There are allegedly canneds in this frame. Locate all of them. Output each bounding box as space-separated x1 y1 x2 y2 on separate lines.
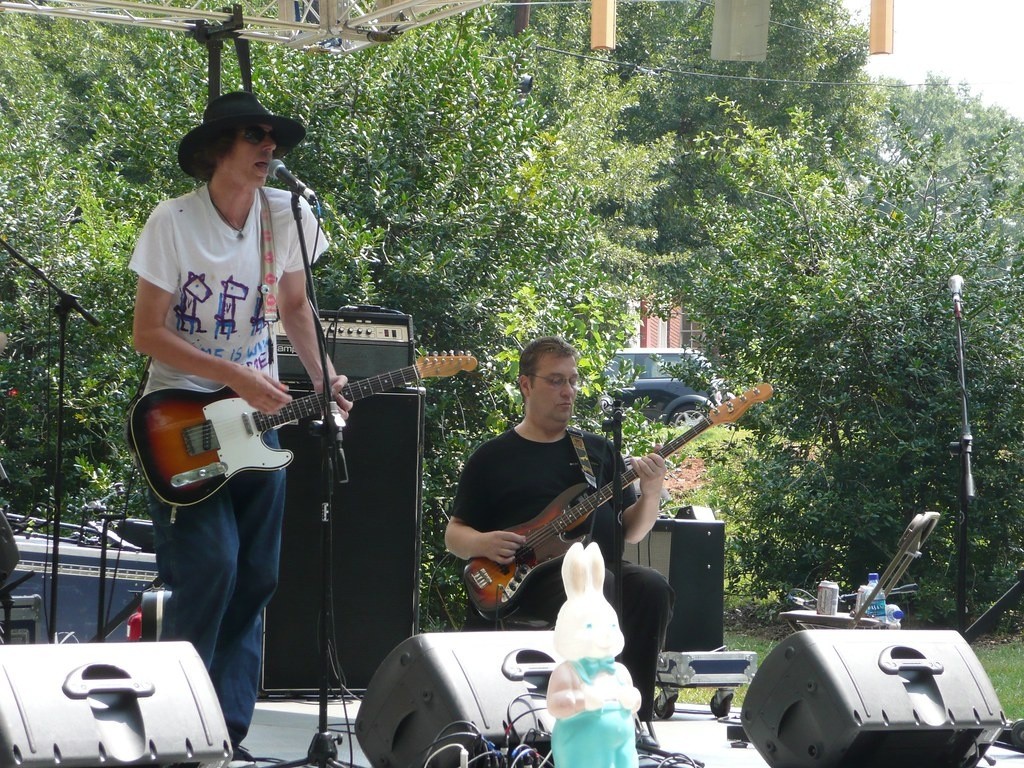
817 581 839 615
856 585 871 617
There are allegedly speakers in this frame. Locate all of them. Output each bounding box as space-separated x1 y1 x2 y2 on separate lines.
258 381 426 699
353 629 561 768
622 505 724 652
0 640 233 768
739 628 1007 768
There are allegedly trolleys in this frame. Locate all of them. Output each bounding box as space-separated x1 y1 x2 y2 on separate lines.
653 646 758 718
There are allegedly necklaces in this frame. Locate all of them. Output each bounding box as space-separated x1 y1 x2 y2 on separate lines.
210 198 254 238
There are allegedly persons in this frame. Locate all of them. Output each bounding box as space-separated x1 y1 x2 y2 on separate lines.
127 92 352 761
445 337 677 748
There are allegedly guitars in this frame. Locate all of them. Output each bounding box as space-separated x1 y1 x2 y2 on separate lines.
462 381 776 623
125 348 479 511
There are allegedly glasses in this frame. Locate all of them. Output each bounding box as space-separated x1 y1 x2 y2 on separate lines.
240 125 278 145
527 372 578 391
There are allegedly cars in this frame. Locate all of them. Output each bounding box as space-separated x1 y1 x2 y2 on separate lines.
601 348 723 430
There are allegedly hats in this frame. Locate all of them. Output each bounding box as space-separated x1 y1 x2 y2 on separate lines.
178 91 306 182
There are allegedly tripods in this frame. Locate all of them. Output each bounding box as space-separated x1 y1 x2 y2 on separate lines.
260 193 363 768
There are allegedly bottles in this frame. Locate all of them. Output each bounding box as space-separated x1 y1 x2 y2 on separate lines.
884 602 903 625
860 573 886 627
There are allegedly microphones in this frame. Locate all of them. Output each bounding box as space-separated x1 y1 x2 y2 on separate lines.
598 394 613 409
267 159 317 202
948 274 964 303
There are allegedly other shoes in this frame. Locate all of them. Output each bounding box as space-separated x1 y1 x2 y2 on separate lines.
232 742 255 762
